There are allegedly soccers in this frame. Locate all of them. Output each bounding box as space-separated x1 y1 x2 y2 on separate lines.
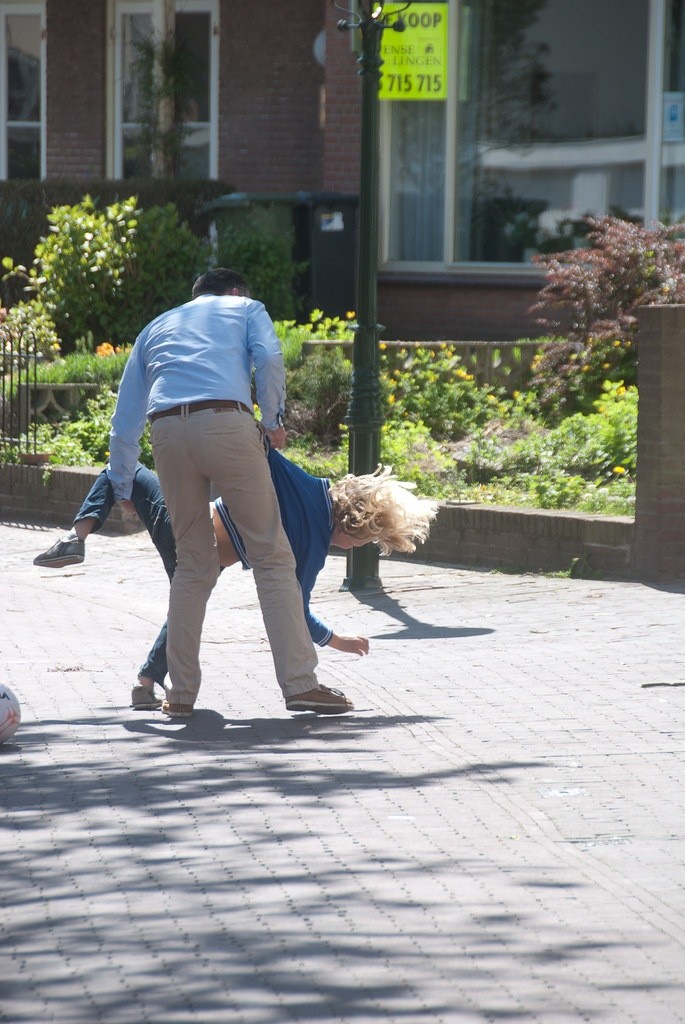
0 680 22 747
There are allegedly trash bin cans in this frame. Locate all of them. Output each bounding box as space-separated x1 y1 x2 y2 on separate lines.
197 190 363 324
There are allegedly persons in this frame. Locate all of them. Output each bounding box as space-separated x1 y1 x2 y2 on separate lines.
108 267 354 716
33 431 436 709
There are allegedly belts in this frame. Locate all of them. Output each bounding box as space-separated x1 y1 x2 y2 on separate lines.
151 400 251 423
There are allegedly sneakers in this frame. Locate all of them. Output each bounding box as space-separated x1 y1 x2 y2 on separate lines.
285 684 355 715
33 537 85 568
162 699 193 718
132 686 163 709
